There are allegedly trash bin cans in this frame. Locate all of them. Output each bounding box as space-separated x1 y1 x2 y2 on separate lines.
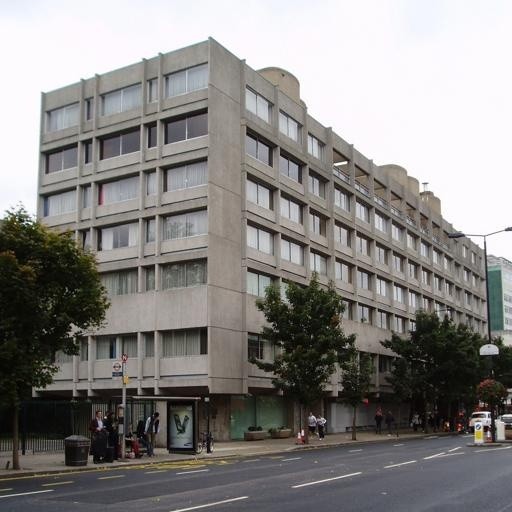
64 435 92 466
495 419 505 440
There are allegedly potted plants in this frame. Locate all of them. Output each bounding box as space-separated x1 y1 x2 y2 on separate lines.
268 425 292 438
244 426 266 441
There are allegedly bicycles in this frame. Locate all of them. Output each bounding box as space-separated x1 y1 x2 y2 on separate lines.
195 432 213 455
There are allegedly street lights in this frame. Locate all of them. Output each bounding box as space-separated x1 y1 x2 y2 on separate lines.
204 396 211 454
447 226 512 442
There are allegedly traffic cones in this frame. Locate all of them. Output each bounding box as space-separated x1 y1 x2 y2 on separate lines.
485 428 493 444
296 432 303 445
301 429 305 442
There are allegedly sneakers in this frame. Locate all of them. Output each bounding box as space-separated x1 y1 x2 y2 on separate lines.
93 459 103 464
317 433 324 440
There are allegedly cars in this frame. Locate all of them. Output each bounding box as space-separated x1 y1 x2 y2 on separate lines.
469 412 492 430
501 413 512 430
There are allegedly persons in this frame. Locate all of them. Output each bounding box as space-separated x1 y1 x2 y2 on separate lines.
136 416 151 456
103 410 109 429
315 413 326 440
89 410 107 463
374 407 477 434
107 411 120 460
143 411 161 456
308 411 317 439
